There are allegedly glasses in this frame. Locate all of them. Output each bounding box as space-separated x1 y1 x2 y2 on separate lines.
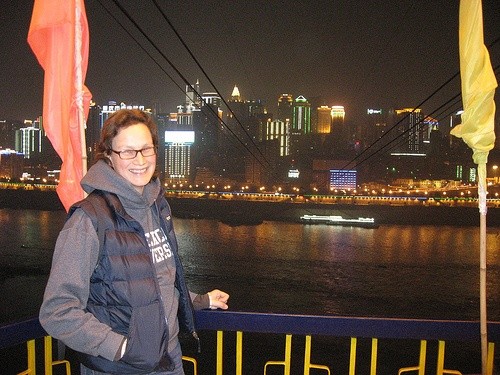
110 147 158 160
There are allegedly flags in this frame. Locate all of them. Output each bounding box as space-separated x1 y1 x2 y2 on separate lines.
24 1 94 215
449 0 499 163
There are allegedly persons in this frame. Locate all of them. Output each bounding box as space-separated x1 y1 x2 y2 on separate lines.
38 110 231 375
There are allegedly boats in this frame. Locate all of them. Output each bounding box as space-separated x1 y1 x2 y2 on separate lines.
298 214 379 229
